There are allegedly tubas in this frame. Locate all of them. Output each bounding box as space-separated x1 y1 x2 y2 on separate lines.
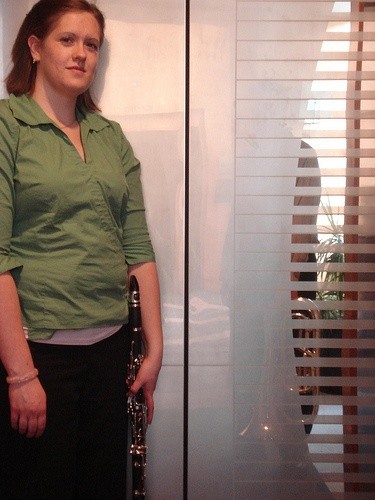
288 296 320 424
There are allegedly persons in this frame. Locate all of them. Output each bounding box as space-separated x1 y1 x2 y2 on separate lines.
291 139 322 436
1 2 162 500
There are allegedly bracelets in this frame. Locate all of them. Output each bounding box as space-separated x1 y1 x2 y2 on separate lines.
6 369 39 385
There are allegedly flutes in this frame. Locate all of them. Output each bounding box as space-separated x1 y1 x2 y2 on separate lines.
126 274 148 500
